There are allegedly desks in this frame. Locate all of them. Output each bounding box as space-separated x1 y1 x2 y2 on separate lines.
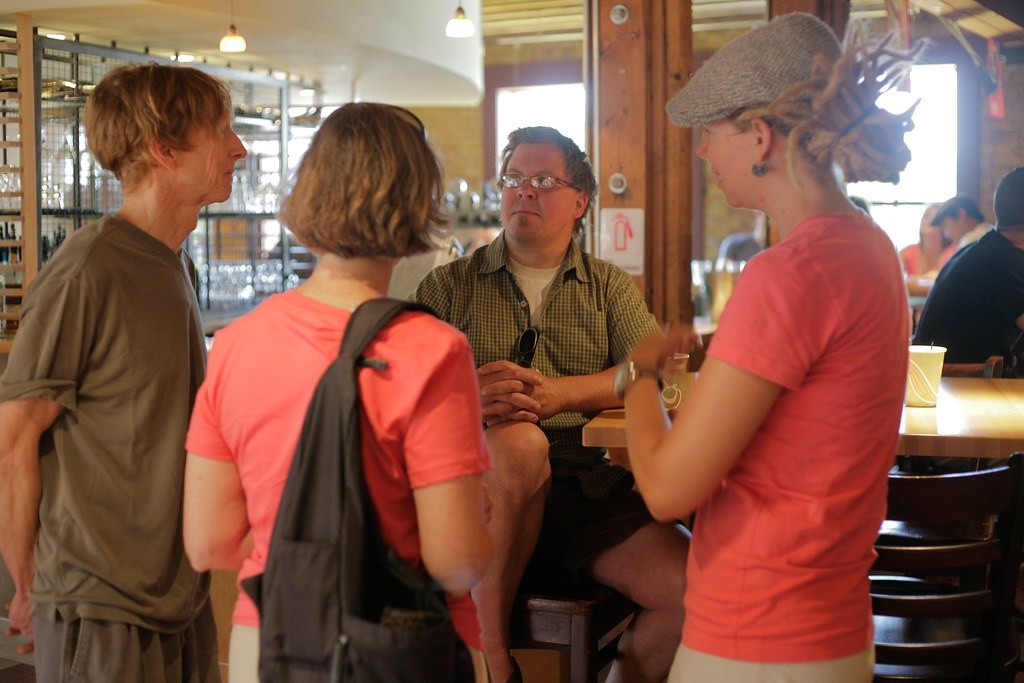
584 369 1024 462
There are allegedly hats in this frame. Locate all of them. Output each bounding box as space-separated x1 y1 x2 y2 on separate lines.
666 13 841 127
993 166 1024 230
930 197 974 226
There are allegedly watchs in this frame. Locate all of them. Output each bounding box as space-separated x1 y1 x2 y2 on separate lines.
614 360 663 400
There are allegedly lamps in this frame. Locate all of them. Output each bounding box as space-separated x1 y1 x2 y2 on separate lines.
443 0 476 40
218 0 247 53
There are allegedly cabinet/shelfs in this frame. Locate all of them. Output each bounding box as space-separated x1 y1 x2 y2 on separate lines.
0 23 315 353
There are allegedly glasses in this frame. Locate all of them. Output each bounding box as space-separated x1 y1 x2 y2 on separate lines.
517 327 540 369
501 174 581 191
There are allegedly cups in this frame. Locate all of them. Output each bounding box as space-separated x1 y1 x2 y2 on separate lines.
658 351 690 406
904 345 947 406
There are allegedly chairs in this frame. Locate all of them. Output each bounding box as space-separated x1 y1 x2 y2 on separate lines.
864 448 1024 683
509 578 636 683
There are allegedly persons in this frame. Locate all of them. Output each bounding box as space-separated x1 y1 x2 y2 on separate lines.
184 105 493 683
408 125 686 682
717 166 1024 643
614 13 912 683
0 62 250 682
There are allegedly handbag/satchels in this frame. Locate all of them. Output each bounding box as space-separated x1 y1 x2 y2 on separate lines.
240 297 474 683
